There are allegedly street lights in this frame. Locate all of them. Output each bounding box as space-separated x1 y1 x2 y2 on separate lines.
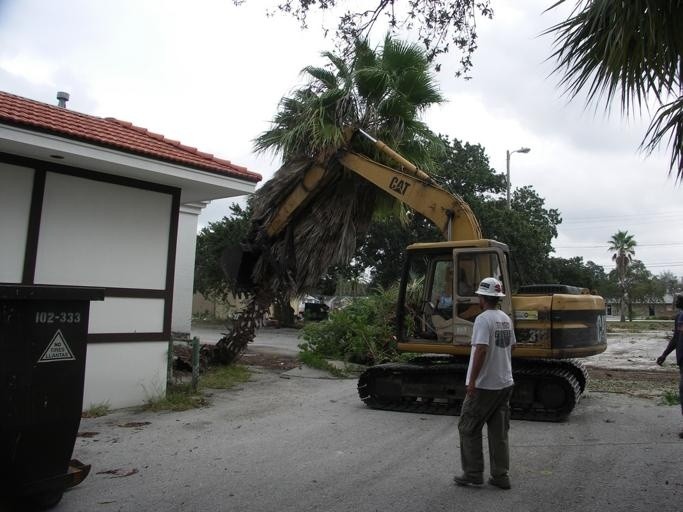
503 147 530 209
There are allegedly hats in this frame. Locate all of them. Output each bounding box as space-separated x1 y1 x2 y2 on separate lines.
472 277 507 297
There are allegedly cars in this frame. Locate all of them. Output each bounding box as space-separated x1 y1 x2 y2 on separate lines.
262 316 279 327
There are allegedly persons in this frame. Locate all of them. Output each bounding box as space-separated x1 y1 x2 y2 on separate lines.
453 278 517 490
656 294 683 438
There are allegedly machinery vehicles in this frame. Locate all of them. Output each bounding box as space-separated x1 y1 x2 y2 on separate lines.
217 117 608 422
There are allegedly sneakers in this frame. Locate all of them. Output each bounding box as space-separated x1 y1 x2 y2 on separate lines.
486 474 513 491
453 469 486 487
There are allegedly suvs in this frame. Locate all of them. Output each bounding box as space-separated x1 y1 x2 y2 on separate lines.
297 299 319 319
